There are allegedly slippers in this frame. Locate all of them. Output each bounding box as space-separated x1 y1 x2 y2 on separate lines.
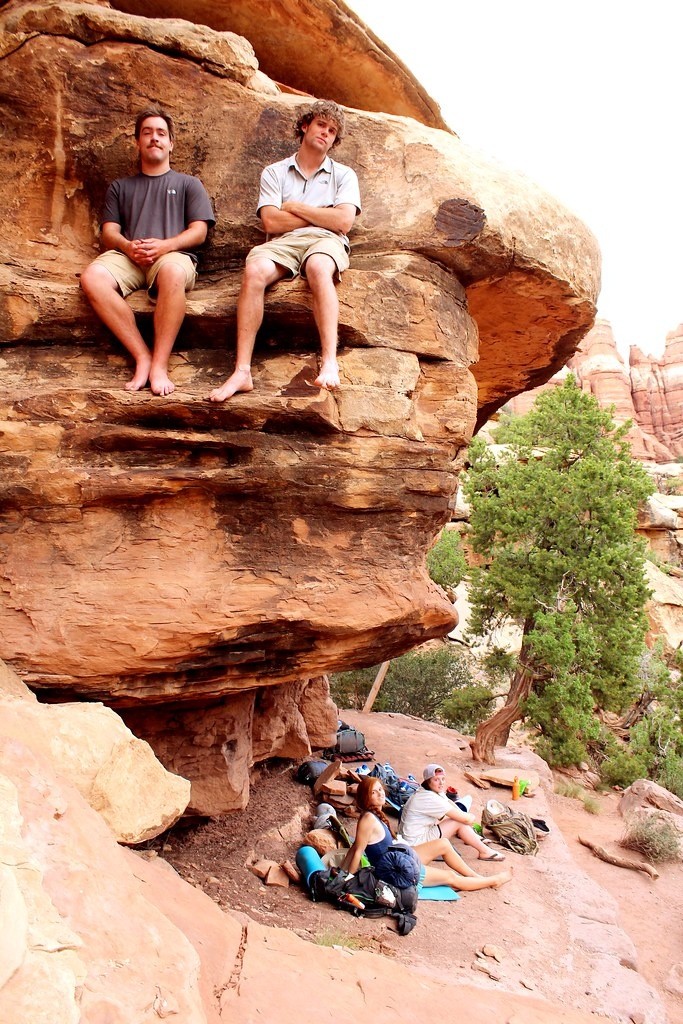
477 851 506 861
464 833 492 845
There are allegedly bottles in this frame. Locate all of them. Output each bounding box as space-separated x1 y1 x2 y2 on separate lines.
384 763 394 773
408 773 415 781
346 893 365 910
360 763 369 775
513 776 519 799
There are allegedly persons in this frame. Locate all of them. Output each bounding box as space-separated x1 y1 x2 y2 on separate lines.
80 105 217 394
210 99 362 402
341 777 515 891
398 764 506 861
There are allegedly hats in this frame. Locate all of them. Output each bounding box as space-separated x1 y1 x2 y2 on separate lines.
423 764 445 781
